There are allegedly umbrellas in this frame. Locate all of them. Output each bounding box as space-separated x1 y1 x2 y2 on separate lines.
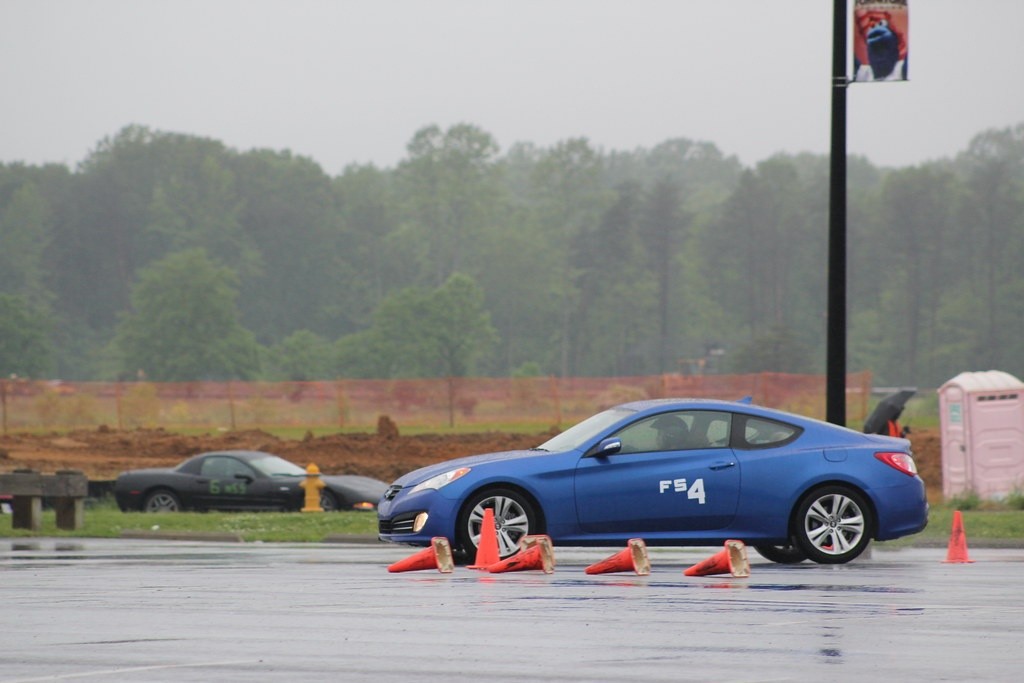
862 388 915 434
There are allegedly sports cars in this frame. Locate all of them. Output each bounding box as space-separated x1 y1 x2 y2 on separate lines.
115 448 390 517
379 390 930 565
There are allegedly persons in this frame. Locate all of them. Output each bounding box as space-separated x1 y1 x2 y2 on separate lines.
648 414 688 451
879 407 910 439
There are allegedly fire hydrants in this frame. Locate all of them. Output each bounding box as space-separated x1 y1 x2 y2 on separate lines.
298 462 326 514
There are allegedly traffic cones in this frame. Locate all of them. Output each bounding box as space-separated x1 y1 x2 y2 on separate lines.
937 508 977 565
520 535 557 568
684 540 751 579
386 536 454 573
466 507 501 569
485 537 555 575
583 537 651 576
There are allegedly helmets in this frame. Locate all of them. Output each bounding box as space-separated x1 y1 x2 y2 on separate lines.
650 414 690 440
